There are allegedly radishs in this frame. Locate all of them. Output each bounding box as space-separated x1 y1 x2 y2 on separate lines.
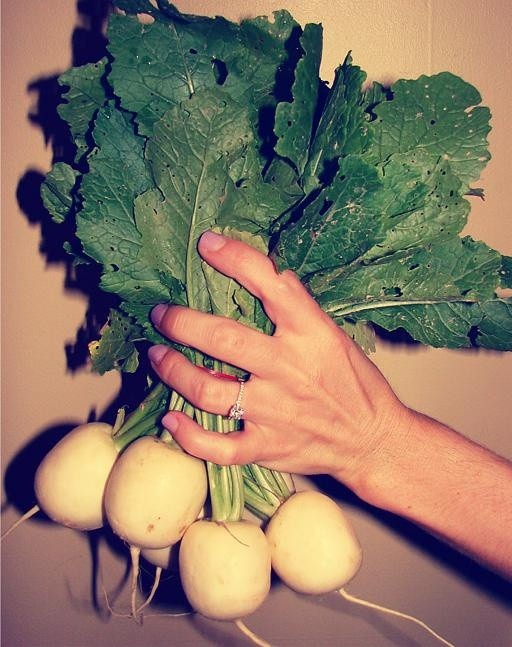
104 436 209 619
178 519 270 647
0 421 120 542
264 491 454 647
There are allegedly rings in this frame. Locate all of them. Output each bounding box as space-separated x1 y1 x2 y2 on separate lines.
225 379 246 423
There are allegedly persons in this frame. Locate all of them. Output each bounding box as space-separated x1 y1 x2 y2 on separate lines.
146 229 512 586
